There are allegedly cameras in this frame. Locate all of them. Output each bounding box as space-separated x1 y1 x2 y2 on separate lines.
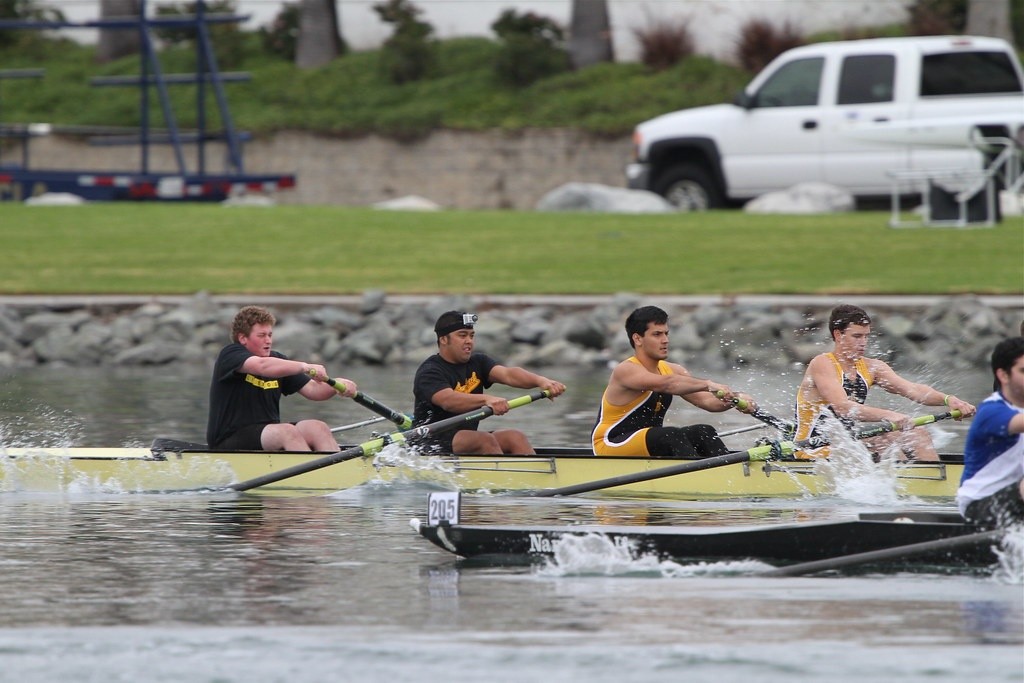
462 313 478 325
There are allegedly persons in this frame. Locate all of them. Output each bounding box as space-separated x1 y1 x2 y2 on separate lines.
205 304 358 452
411 309 567 455
591 305 760 457
956 337 1024 528
792 304 977 462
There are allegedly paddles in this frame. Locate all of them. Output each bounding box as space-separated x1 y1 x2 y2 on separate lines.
409 405 976 496
563 522 1023 578
306 367 418 431
127 383 568 491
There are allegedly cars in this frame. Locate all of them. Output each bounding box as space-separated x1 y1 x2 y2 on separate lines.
623 35 1024 215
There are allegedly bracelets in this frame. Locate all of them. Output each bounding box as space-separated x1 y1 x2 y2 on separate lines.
944 395 950 406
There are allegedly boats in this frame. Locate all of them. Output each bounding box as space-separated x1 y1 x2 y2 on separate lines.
1 434 963 500
407 508 997 572
